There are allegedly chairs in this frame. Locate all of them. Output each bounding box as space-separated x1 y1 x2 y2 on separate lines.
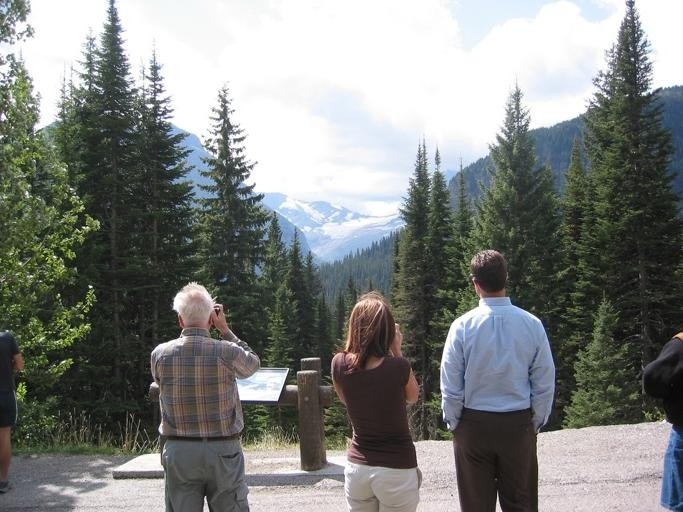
1 481 10 492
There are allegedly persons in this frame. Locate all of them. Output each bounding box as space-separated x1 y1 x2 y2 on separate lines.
331 288 422 512
642 332 683 512
0 332 25 493
150 281 261 512
438 248 557 511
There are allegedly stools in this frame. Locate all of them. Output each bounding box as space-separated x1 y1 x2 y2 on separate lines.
166 435 242 441
